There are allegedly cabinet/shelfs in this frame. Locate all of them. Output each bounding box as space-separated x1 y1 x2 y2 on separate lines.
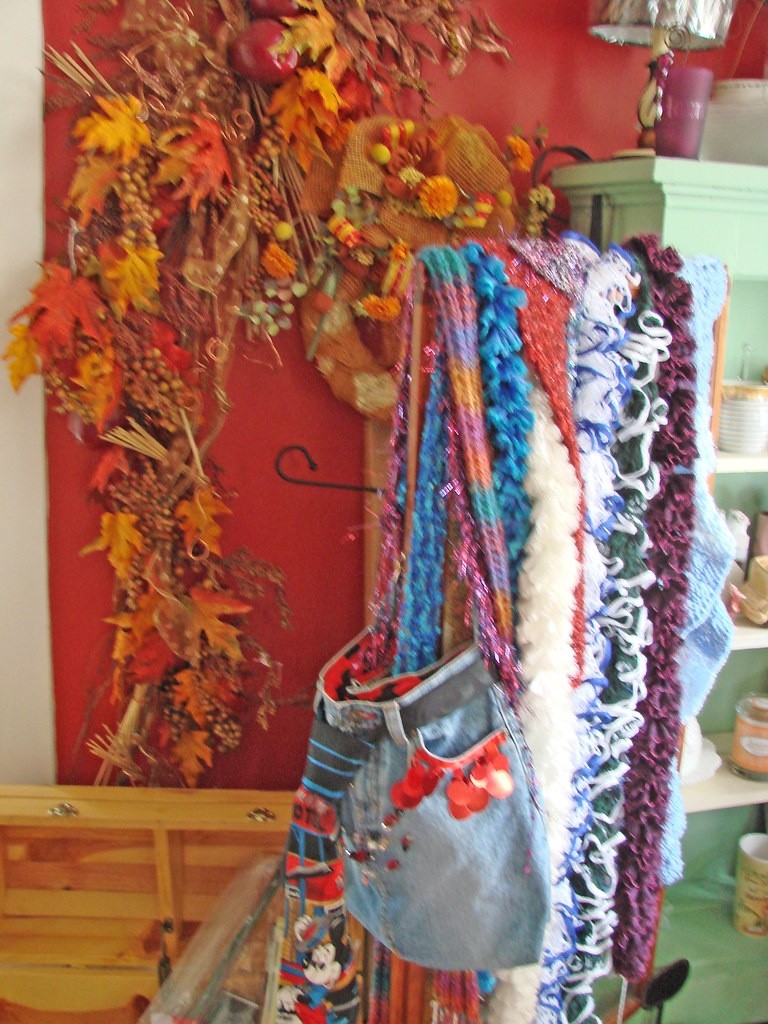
551 152 768 1024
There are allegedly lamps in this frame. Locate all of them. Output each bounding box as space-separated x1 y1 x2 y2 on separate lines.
587 0 738 159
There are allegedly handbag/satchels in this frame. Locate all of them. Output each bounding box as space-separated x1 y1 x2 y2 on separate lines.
300 111 520 423
276 621 551 1024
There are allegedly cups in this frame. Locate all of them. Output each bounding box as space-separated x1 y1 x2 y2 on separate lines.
718 379 768 455
754 511 768 558
655 64 714 159
733 833 768 938
715 77 766 102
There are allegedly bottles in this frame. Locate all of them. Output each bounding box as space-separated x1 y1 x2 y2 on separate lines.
728 511 751 571
729 693 768 782
461 968 499 1024
